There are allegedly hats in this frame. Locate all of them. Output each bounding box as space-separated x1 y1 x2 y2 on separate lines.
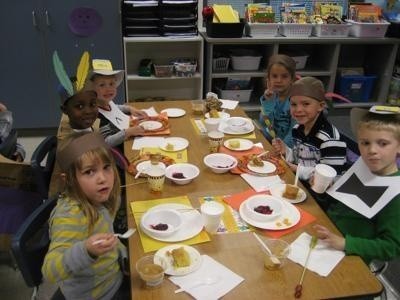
88 59 125 88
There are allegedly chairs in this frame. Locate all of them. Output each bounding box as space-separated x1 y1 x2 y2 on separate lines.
32 134 60 197
338 129 362 174
10 197 62 299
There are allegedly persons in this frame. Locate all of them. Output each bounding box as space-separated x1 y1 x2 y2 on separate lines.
47 75 128 206
259 53 297 143
307 113 400 266
40 131 131 299
270 76 347 181
88 58 148 148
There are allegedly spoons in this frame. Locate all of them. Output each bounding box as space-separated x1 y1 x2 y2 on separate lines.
174 276 221 294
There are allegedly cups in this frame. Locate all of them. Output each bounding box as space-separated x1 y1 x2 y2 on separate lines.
136 256 168 286
261 238 290 271
312 162 338 195
191 100 205 113
200 201 224 233
147 167 165 192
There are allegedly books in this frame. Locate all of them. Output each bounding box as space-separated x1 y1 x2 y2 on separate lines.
211 1 384 26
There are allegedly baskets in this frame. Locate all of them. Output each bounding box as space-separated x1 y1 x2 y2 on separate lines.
213 58 230 71
245 19 391 37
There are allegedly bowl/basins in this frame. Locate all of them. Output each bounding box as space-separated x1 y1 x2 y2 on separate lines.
204 153 238 175
245 196 282 221
145 210 183 234
166 163 202 185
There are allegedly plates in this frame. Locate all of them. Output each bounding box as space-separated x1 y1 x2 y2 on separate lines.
240 194 299 232
205 110 255 153
136 161 165 176
269 182 306 204
247 159 276 172
155 244 202 277
138 107 189 151
141 203 203 242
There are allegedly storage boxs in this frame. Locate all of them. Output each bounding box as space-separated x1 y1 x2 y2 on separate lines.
207 19 244 38
344 18 390 37
282 53 309 70
339 73 378 102
216 86 254 103
229 54 263 71
312 22 350 36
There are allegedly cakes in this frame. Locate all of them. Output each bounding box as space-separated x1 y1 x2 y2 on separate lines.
171 246 190 268
165 141 174 150
209 108 220 119
228 140 240 148
282 185 299 200
254 157 265 168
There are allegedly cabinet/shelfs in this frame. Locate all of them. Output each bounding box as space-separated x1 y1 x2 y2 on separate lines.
202 29 400 113
123 37 203 100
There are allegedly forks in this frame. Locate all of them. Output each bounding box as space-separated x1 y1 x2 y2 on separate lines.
93 227 137 246
